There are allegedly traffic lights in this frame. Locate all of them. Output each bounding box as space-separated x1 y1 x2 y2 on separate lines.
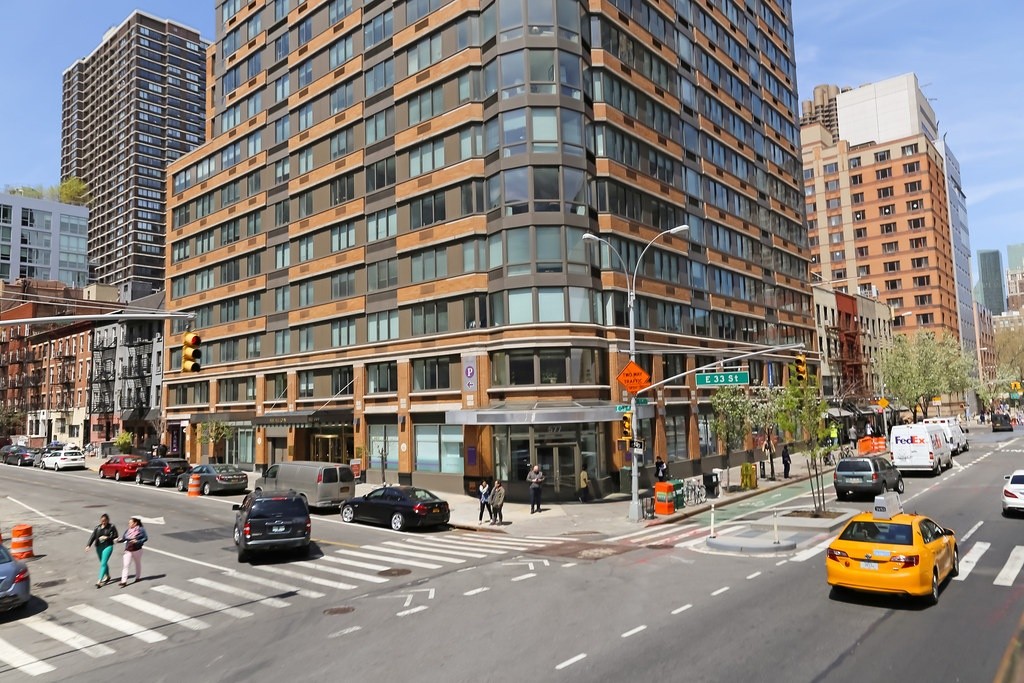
180 329 203 374
621 413 632 438
794 354 808 383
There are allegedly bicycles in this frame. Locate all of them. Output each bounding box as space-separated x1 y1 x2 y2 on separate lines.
684 479 709 507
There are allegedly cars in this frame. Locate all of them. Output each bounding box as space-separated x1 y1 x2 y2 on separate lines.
338 485 452 532
0 443 87 471
833 456 904 502
1001 469 1024 518
231 488 312 563
98 454 148 481
175 463 249 496
135 458 193 487
824 491 959 607
0 545 32 617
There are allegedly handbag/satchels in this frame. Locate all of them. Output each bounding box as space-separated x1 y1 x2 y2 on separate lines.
658 468 663 477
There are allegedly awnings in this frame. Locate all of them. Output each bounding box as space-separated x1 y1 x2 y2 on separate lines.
251 409 353 428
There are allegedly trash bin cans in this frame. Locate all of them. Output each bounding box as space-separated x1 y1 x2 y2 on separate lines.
741 463 758 489
654 481 675 514
702 472 719 497
670 480 685 509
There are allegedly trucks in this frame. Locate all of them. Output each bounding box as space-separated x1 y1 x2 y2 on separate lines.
254 460 355 515
923 417 970 455
890 422 954 476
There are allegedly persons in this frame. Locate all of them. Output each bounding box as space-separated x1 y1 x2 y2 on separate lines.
579 463 590 504
830 421 874 450
761 438 775 462
478 480 493 524
655 456 667 481
117 518 148 586
526 465 545 514
489 480 505 525
782 445 792 478
85 513 118 587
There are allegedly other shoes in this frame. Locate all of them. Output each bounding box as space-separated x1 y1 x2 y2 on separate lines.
531 508 534 514
496 522 503 525
579 497 583 504
134 577 139 583
118 582 126 586
478 520 481 525
489 521 496 525
102 576 110 582
537 508 541 512
95 584 101 588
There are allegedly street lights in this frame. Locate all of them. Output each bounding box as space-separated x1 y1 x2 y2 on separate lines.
875 310 913 444
581 225 690 524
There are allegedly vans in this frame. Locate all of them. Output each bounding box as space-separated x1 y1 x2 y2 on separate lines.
992 413 1013 432
